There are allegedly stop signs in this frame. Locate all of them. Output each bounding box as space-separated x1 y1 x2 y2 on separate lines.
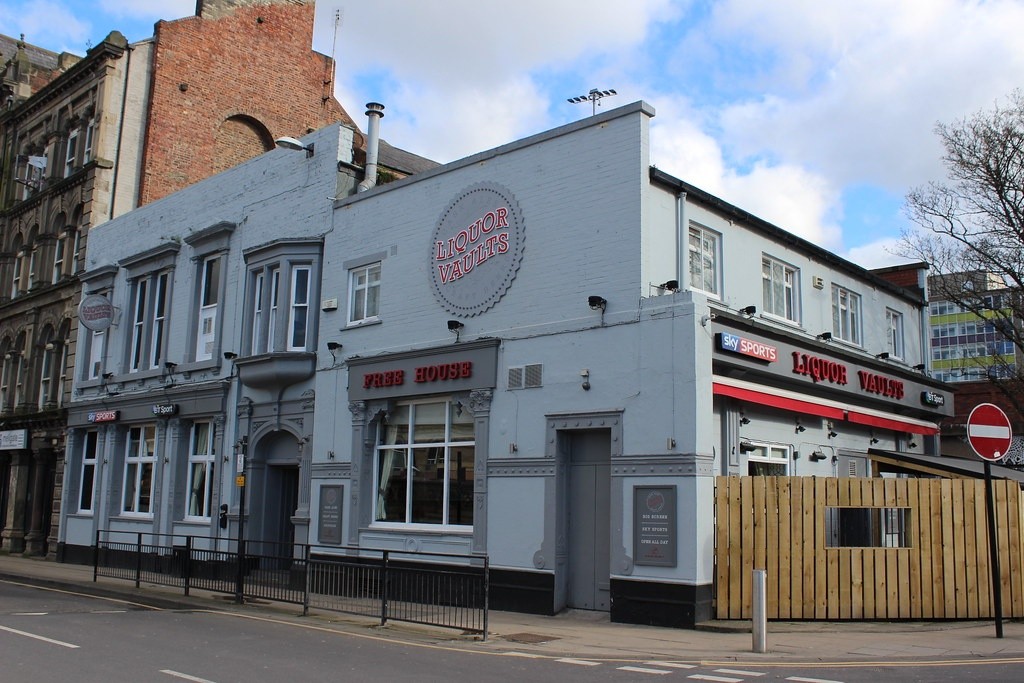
966 403 1012 461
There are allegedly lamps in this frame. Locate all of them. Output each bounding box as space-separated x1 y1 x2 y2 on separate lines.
660 280 679 291
740 418 750 426
447 320 464 333
165 361 178 369
588 296 607 310
818 333 831 342
224 352 237 359
102 373 114 380
796 425 806 434
913 364 925 371
740 306 756 317
812 451 826 460
876 352 889 360
870 438 879 444
910 443 917 448
275 136 314 152
327 342 343 351
828 432 837 439
740 442 756 454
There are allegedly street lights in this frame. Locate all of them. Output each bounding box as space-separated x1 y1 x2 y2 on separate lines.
233 435 248 603
567 89 617 117
